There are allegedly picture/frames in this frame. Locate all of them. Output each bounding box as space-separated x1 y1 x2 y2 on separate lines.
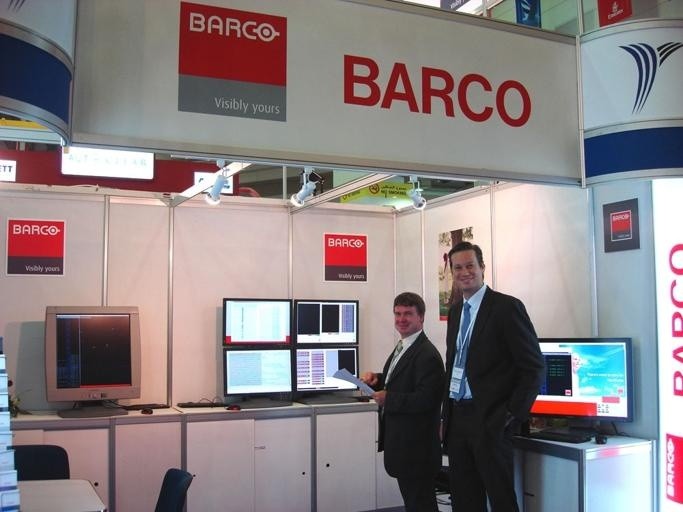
601 197 641 254
321 230 370 285
4 215 66 278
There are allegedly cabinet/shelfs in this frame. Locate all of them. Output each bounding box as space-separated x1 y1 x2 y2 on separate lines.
314 410 379 511
115 420 182 512
515 425 658 512
12 428 43 443
42 428 112 511
253 415 314 512
185 419 253 512
377 449 406 509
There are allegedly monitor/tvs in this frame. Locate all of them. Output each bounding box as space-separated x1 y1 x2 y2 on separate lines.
528 338 633 435
294 299 358 345
46 306 141 418
293 345 359 405
223 349 293 408
222 298 293 349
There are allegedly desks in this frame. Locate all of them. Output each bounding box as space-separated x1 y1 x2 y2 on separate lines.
12 478 108 511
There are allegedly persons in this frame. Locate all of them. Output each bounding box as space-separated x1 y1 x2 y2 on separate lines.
362 290 446 511
442 247 543 511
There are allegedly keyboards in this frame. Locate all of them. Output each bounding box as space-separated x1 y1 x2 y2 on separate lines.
526 432 592 443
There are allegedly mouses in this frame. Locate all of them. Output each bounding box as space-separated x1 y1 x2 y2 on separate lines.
143 407 154 415
595 435 607 444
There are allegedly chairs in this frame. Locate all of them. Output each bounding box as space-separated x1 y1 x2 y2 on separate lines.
7 444 70 479
154 466 193 511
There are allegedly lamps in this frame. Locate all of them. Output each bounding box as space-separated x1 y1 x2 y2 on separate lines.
205 156 429 211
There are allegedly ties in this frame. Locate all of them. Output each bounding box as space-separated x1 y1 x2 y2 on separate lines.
379 340 403 418
451 301 471 403
